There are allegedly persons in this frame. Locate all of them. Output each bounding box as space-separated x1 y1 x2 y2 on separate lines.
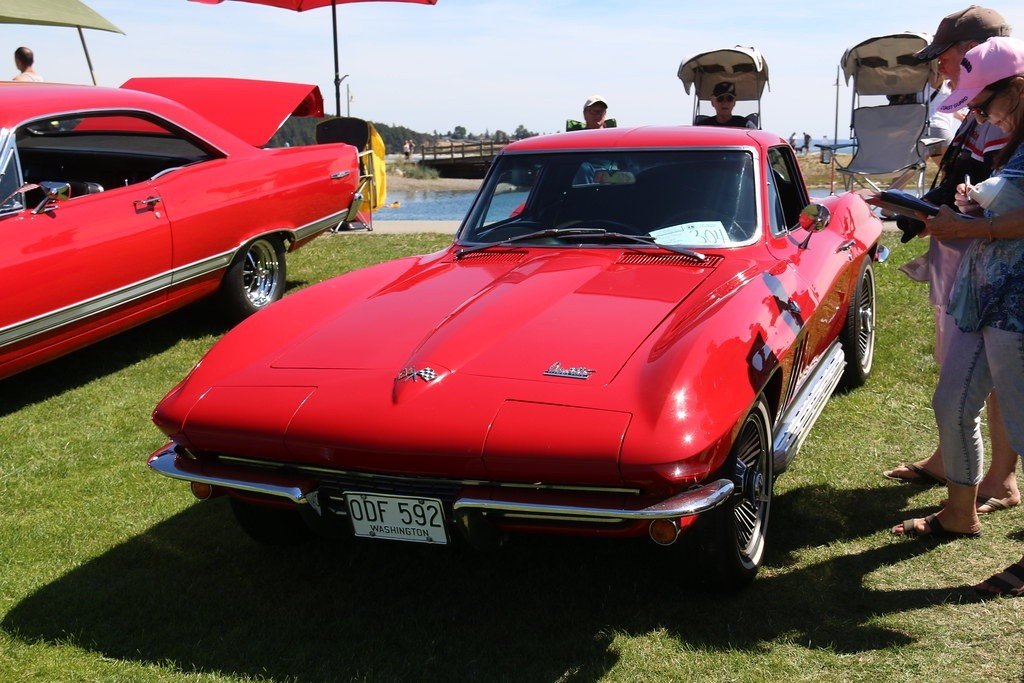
789 132 796 149
892 35 1024 597
403 139 411 158
882 4 1021 515
696 82 757 130
801 132 810 153
408 140 415 156
12 47 44 82
571 97 618 186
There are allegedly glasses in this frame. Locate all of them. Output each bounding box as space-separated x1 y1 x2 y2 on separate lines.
968 89 997 118
585 110 606 115
713 95 735 102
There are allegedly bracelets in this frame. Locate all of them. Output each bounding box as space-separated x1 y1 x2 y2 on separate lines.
989 217 993 243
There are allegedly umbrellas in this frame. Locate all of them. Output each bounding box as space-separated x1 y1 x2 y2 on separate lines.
188 0 438 117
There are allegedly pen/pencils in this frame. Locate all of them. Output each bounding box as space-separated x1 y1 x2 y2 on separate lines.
964 174 971 203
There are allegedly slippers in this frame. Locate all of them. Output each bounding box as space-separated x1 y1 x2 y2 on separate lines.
882 464 946 484
941 492 1010 513
891 513 982 537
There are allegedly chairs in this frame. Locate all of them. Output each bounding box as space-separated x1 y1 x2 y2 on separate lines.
566 118 616 132
812 58 932 214
692 65 763 130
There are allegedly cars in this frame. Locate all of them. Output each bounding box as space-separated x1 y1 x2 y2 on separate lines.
0 77 362 389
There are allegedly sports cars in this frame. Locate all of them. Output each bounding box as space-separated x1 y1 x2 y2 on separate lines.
142 123 892 595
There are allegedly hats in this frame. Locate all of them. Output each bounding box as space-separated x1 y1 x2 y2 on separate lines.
913 3 1014 67
583 95 608 109
935 36 1024 113
712 82 736 97
